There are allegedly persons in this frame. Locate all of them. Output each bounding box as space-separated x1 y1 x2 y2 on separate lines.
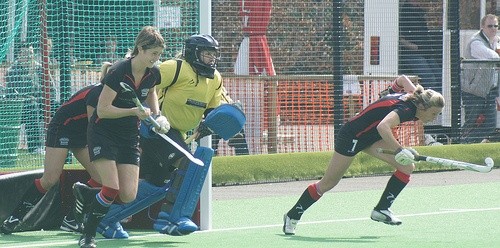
72 25 171 248
398 0 442 92
96 34 246 239
0 37 58 154
460 14 500 144
283 75 445 236
0 57 119 235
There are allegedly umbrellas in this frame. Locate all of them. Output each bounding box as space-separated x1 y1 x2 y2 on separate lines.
236 0 280 133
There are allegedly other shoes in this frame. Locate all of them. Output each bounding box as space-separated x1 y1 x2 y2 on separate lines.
33 149 45 156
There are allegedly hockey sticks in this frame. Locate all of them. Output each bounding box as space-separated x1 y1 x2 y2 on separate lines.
167 131 199 160
151 127 204 167
120 81 160 129
376 148 494 173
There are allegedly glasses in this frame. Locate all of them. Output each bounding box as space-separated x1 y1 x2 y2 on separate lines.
484 23 499 29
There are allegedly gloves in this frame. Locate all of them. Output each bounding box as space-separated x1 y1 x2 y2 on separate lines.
395 147 415 165
154 116 170 134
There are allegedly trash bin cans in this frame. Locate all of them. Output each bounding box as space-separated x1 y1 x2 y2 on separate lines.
0 98 26 168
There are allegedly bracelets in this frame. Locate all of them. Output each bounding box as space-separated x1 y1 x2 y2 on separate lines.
153 110 162 119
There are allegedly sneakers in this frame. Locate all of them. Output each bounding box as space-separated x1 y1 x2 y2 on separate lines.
1 216 20 236
71 182 89 222
371 208 402 225
283 213 299 235
60 216 85 236
78 232 96 248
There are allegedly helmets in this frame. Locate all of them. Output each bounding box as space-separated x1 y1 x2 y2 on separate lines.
185 34 220 79
